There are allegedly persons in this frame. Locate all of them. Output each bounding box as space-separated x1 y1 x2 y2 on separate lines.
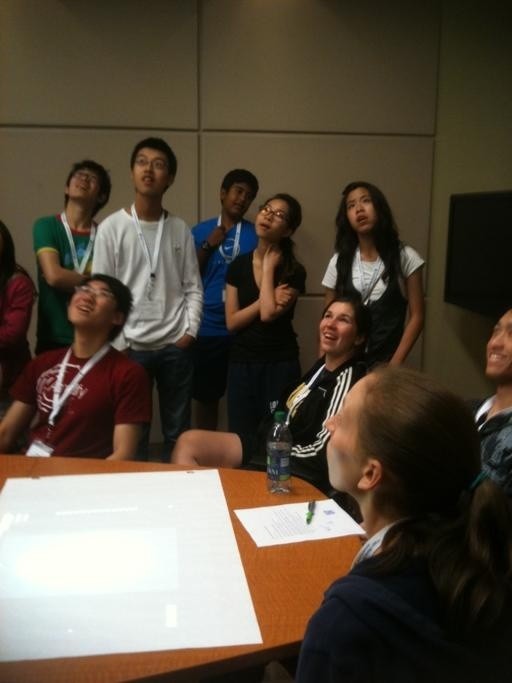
319 181 425 376
263 362 510 682
171 293 369 520
34 159 111 353
223 191 308 435
0 272 153 462
189 169 259 432
92 133 204 463
472 303 512 478
0 219 36 455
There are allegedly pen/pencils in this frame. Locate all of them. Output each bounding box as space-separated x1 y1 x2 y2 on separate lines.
306 501 316 524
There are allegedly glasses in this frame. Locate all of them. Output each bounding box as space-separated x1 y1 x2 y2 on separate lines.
73 171 101 181
260 206 286 218
72 284 117 300
136 159 167 168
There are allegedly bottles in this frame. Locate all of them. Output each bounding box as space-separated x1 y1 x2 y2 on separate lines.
266 412 291 494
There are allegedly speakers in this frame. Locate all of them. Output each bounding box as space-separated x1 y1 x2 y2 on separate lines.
444 191 512 318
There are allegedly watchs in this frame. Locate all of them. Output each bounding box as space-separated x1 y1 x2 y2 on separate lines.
201 240 212 252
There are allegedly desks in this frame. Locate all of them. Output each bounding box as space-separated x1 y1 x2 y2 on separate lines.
1 453 369 683
